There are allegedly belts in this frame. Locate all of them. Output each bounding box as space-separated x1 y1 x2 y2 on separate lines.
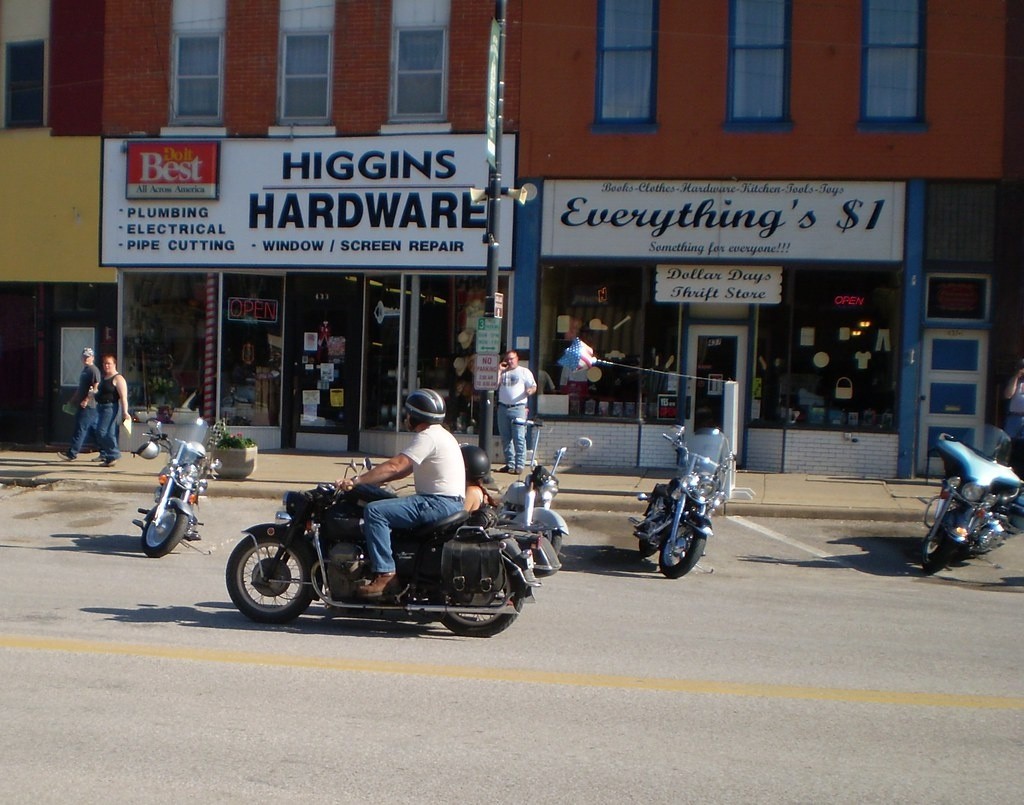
1009 412 1024 416
500 403 525 408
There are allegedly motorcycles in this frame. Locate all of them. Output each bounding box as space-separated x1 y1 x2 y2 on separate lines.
224 417 564 638
627 424 735 580
129 408 222 559
917 422 1024 576
499 418 570 557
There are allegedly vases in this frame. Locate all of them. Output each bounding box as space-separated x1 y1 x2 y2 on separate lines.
213 446 258 478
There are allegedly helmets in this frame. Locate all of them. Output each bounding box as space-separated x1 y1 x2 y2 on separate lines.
405 388 446 426
135 439 161 459
460 446 491 484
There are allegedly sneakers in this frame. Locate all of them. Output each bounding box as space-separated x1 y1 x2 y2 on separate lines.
359 573 398 596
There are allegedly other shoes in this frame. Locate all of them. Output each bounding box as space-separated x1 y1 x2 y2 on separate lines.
515 467 523 474
57 451 73 461
100 459 118 467
500 466 515 475
92 455 107 462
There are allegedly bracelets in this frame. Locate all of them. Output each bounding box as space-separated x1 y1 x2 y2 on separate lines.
351 476 360 486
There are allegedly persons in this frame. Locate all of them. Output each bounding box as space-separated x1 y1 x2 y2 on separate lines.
335 389 466 595
1004 357 1024 439
538 370 588 413
57 346 100 462
460 445 490 513
497 351 537 476
90 354 131 466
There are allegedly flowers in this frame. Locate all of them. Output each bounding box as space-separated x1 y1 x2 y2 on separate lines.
217 432 255 450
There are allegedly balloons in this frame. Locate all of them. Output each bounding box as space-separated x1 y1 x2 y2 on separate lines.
557 337 598 373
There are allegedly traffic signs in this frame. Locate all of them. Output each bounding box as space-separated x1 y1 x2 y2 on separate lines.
494 291 504 319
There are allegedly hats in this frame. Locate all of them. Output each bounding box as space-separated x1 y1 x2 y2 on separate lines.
82 347 94 357
